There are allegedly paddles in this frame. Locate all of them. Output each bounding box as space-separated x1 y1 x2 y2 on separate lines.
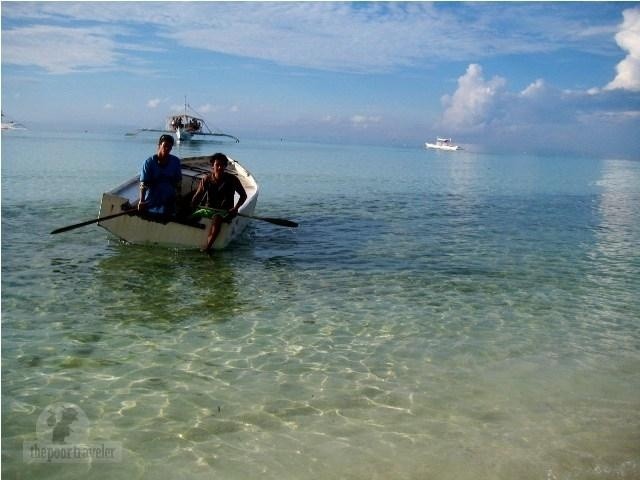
198 204 298 228
50 206 139 235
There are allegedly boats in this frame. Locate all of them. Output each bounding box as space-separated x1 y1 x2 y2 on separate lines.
97 154 259 251
125 97 240 142
425 136 461 151
1 111 29 131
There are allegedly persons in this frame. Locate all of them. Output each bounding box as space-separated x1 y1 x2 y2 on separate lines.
137 135 183 222
187 153 247 251
170 117 202 137
